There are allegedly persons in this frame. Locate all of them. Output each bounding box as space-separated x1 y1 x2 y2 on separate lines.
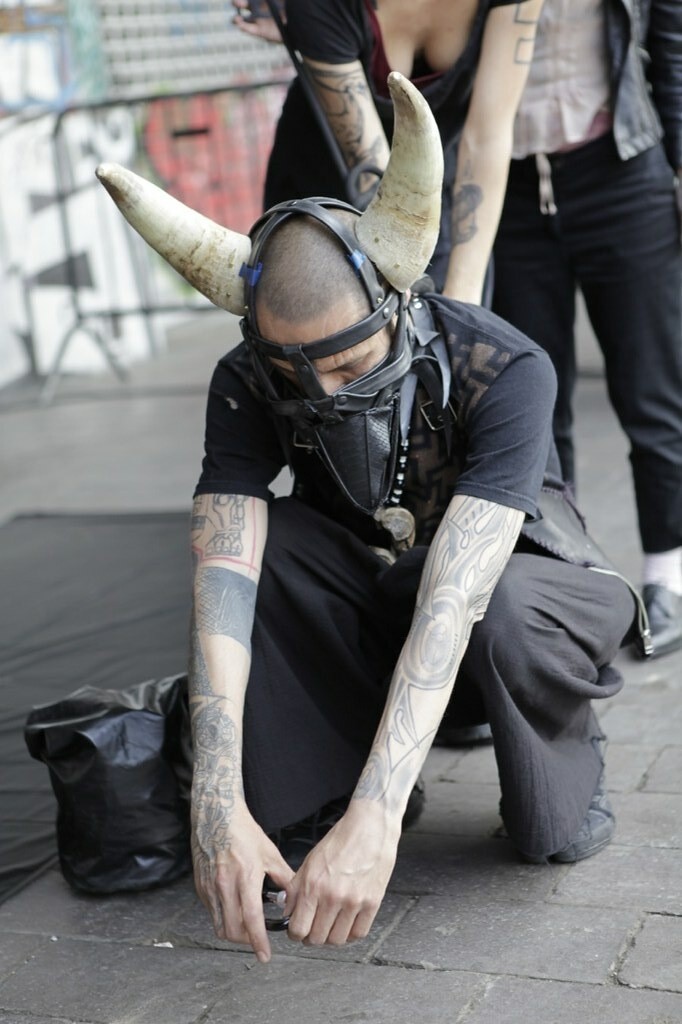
92 72 657 963
228 0 682 659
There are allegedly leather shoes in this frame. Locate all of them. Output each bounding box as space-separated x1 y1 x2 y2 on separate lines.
637 584 682 663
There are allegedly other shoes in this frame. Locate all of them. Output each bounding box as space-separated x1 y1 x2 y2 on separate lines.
257 771 424 899
546 761 616 864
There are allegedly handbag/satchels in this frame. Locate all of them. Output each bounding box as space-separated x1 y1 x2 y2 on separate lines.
24 669 193 896
